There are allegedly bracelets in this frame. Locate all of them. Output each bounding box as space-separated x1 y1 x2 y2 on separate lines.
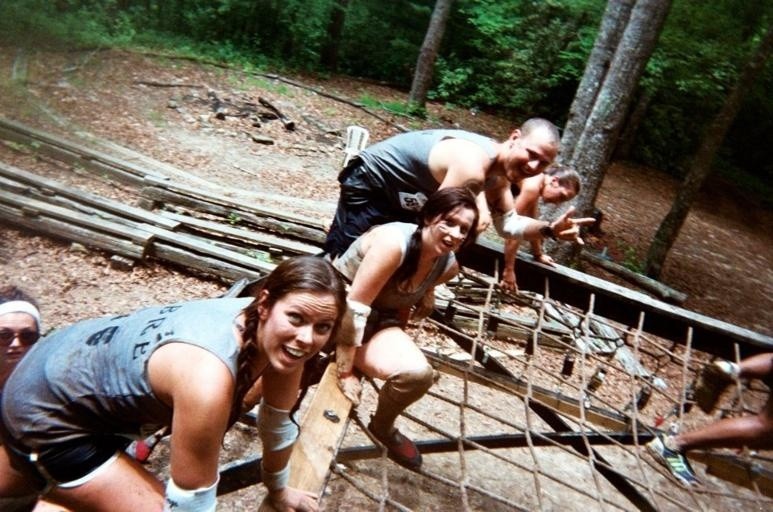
258 459 290 490
542 220 555 240
336 363 353 381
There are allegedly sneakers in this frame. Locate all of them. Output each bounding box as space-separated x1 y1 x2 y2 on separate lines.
638 432 704 488
366 415 422 468
693 359 742 415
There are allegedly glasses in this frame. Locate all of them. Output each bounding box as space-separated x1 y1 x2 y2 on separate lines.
0 331 38 345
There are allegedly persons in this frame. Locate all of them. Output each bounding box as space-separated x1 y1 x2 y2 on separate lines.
0 255 348 512
320 116 597 259
0 283 85 512
455 171 584 296
643 352 773 490
239 183 479 472
